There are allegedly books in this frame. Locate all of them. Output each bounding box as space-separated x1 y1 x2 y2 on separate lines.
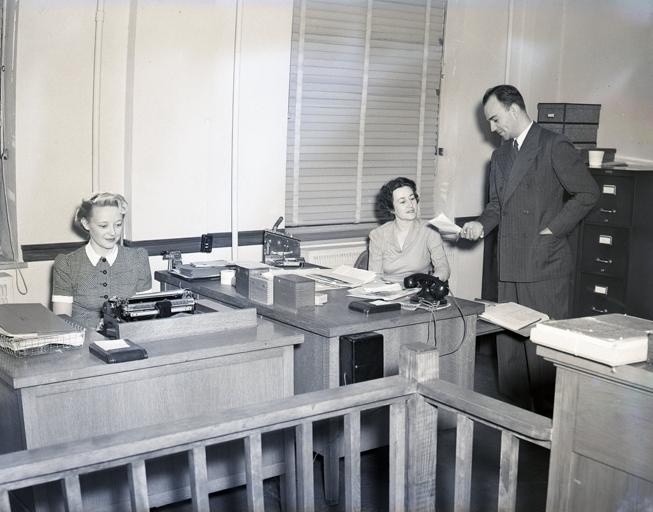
169 257 234 281
268 262 419 312
529 310 652 368
470 295 551 341
1 302 87 360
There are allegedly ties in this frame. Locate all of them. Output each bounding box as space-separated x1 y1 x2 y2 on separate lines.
511 140 519 164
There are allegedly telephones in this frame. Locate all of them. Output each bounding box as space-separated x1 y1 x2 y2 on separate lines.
404 273 449 307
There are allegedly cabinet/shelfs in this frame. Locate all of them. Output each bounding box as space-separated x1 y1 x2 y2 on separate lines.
480 156 652 327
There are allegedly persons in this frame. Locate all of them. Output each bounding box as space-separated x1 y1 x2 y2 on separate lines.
365 174 451 282
458 83 600 419
50 191 153 330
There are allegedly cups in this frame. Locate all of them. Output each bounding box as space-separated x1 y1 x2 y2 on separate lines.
587 150 604 168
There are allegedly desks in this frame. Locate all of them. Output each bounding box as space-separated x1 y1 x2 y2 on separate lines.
1 301 306 510
536 332 653 512
155 255 486 458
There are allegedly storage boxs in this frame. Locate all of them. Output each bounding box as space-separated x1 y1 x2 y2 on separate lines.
570 142 597 160
535 100 601 126
537 121 599 145
272 274 316 312
232 261 269 297
